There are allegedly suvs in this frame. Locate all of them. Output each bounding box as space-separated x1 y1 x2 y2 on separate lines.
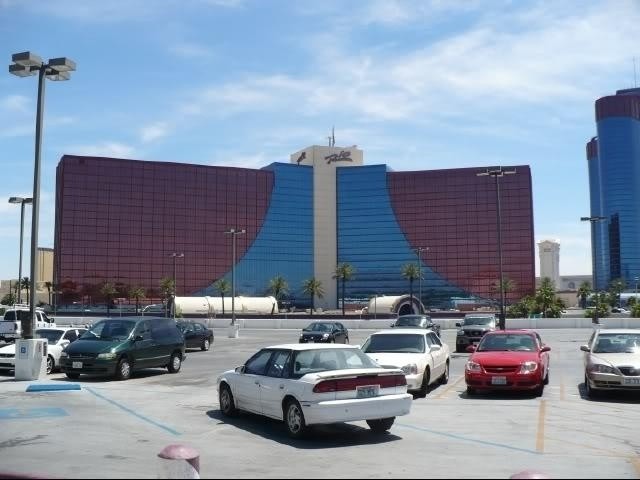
455 313 502 352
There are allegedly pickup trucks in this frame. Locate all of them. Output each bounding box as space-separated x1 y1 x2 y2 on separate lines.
0 305 57 341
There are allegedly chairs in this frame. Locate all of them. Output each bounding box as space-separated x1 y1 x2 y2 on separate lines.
598 339 640 352
110 328 127 337
281 360 336 377
318 325 327 331
193 326 200 331
488 338 533 347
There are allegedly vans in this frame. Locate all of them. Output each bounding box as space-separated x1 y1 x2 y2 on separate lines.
59 317 187 380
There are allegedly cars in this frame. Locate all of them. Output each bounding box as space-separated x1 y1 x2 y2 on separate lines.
217 344 411 437
349 329 449 398
0 326 101 374
299 321 349 343
175 321 214 352
463 330 552 395
391 314 440 339
581 328 640 397
128 303 161 312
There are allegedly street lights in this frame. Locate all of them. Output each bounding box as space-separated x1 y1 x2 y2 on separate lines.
5 52 83 341
633 277 640 305
169 253 185 297
580 215 610 311
7 196 34 303
477 166 518 331
411 245 430 313
223 228 247 324
